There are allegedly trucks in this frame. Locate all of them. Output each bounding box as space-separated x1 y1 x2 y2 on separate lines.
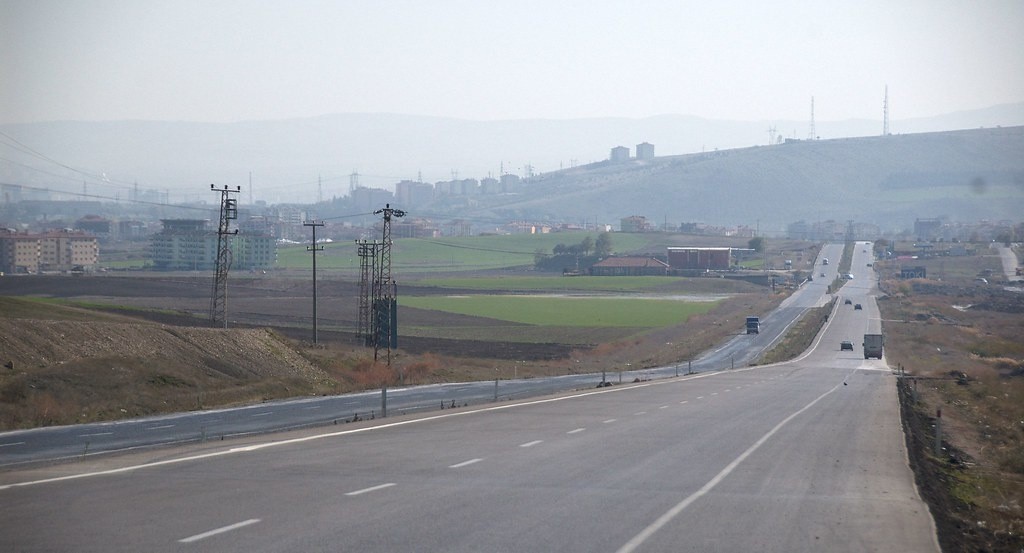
862 334 886 360
744 317 761 334
824 259 828 264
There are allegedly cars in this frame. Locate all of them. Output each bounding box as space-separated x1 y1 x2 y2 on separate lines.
840 341 855 350
821 273 826 277
845 299 853 304
855 304 863 310
974 277 988 284
863 249 866 252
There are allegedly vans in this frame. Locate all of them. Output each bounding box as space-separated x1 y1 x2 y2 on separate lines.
843 274 853 279
